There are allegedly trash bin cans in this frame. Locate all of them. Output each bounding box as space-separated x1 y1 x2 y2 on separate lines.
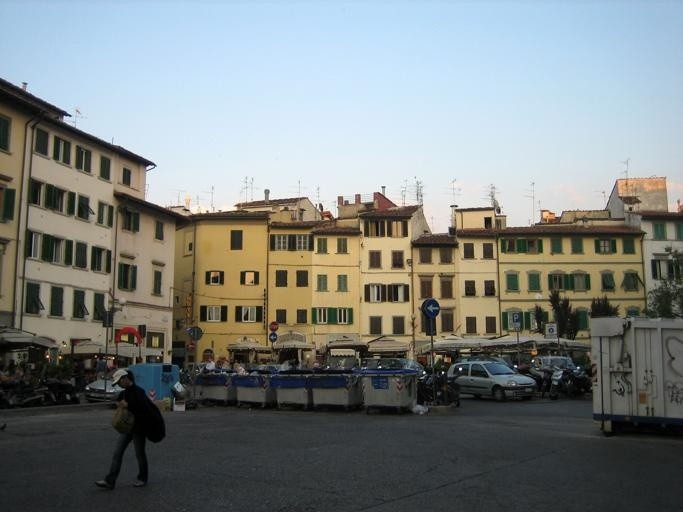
272 372 310 410
310 368 363 411
233 373 277 408
359 369 418 415
196 370 236 406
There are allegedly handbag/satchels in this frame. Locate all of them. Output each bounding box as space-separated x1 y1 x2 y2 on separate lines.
112 406 134 435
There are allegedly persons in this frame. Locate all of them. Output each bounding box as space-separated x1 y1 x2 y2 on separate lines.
196 356 308 376
93 368 148 490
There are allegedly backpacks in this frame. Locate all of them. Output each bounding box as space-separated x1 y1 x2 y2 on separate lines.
147 403 166 443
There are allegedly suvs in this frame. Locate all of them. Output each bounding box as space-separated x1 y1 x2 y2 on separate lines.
453 355 518 374
351 357 424 377
524 356 579 391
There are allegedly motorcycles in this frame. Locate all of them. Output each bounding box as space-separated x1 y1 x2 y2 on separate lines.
539 363 590 400
415 371 464 408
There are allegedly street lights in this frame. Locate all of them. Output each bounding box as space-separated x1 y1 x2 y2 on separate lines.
305 354 310 369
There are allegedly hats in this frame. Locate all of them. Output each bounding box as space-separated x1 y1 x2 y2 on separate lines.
112 370 128 387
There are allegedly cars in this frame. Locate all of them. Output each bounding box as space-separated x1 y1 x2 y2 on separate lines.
447 362 537 402
254 364 292 371
82 367 132 404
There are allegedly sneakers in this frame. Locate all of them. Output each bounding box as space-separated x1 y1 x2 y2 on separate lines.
95 480 114 488
133 480 144 487
